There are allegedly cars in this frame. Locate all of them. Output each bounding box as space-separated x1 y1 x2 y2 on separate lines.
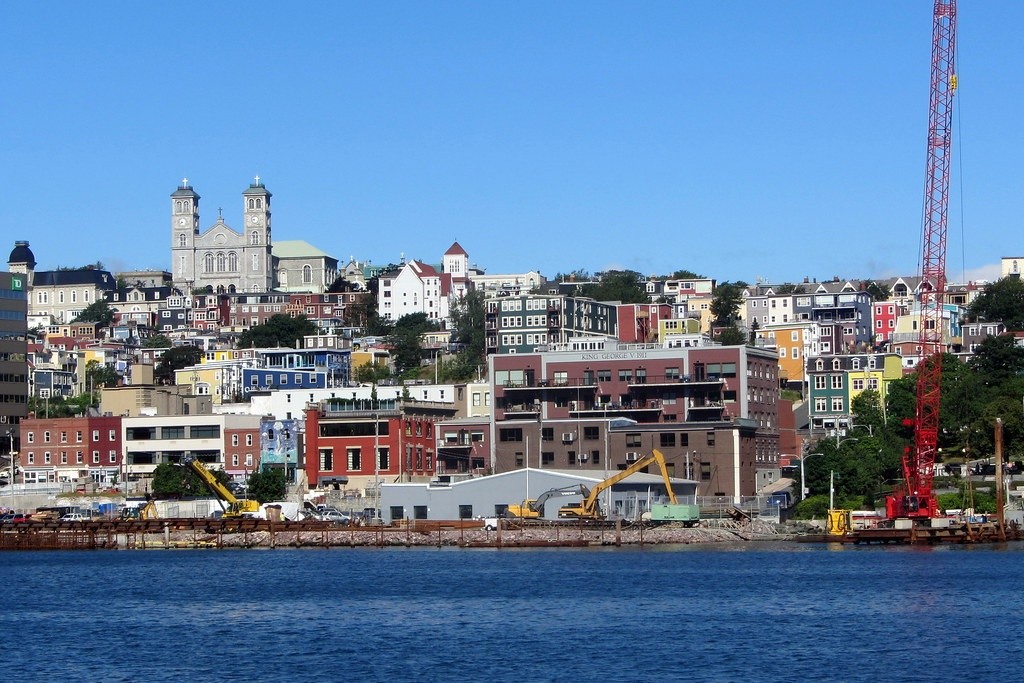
0 514 15 523
316 504 338 512
60 512 82 522
0 465 20 477
322 511 356 525
766 495 784 506
13 513 33 522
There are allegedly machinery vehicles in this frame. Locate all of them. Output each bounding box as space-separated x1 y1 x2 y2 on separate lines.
110 499 159 523
877 0 957 527
558 448 678 520
506 483 601 520
179 457 259 520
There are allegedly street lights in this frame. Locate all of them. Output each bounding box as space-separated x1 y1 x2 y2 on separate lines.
837 438 859 449
853 425 872 435
781 453 824 500
5 428 15 509
436 350 443 384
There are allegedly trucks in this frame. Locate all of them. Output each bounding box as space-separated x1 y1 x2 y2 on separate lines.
642 504 700 527
